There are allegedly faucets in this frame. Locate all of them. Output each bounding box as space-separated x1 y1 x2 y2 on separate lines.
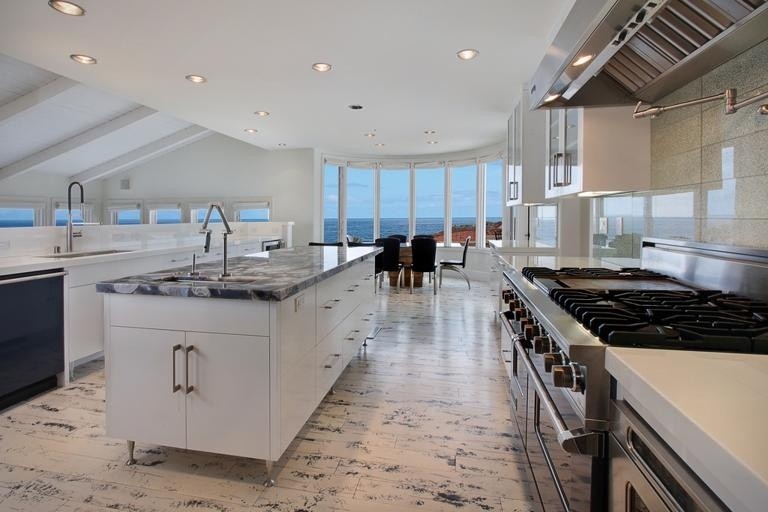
199 204 233 276
67 181 85 252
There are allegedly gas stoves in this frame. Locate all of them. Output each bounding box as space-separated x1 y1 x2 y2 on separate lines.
501 265 767 429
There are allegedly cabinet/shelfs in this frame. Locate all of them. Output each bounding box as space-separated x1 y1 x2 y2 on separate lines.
275 256 378 463
69 241 258 366
502 84 552 208
1 268 69 405
545 104 651 199
488 242 514 378
103 292 281 488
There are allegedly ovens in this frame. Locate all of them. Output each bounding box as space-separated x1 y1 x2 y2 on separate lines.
496 308 609 511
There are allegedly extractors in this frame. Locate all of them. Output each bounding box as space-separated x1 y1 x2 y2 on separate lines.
527 0 767 115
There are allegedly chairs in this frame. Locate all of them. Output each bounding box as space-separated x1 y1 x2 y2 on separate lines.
308 230 475 295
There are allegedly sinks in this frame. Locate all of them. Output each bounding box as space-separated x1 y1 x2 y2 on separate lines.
32 250 135 258
147 272 264 286
